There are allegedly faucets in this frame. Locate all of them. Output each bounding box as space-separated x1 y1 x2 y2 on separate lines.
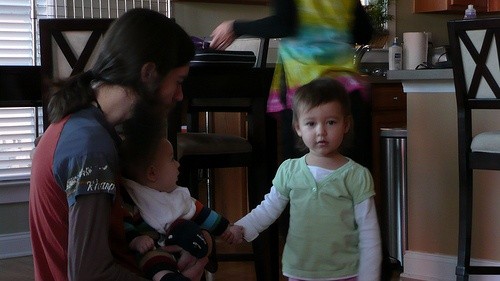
353 44 370 68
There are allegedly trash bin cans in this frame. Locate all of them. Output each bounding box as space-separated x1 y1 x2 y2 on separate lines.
379 126 410 272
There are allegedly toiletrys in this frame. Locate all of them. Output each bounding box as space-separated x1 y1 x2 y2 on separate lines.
389 37 403 71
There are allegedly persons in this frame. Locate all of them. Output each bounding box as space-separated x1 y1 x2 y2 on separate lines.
209 0 374 164
221 79 383 281
29 8 217 281
120 136 244 281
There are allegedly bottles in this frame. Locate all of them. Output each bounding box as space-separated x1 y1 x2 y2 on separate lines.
465 5 476 18
388 37 402 70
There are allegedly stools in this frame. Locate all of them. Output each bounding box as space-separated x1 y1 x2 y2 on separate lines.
447 18 500 280
168 51 266 262
40 18 115 133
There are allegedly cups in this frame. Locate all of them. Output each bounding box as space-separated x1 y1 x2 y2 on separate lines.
403 32 429 70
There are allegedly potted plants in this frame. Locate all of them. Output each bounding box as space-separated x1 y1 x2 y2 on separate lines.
359 0 395 49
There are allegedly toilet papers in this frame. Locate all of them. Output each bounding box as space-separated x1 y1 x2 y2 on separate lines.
403 33 429 70
